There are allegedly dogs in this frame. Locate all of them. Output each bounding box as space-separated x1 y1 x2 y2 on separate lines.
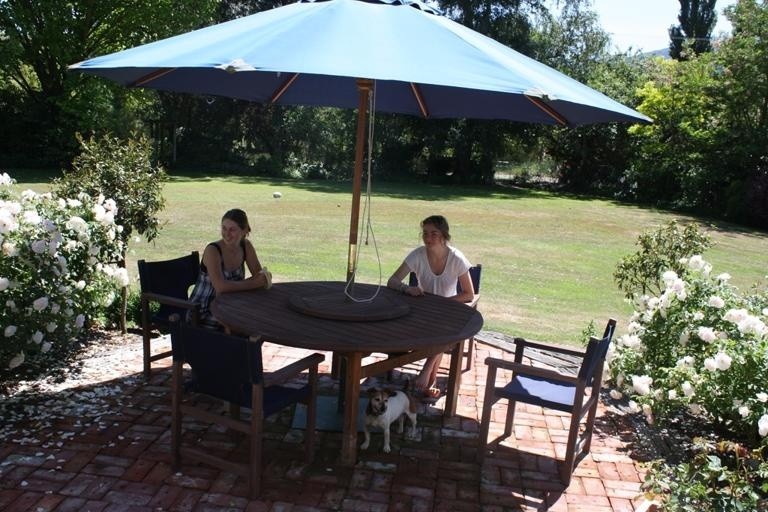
360 387 417 453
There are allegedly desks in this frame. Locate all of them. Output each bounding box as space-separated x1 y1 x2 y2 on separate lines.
210 281 483 470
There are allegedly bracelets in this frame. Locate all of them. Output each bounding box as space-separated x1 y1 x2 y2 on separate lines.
401 284 408 293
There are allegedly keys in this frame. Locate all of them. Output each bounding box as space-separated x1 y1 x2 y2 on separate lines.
65 0 653 300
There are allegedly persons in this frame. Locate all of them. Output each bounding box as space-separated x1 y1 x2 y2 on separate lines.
185 208 266 336
386 214 476 398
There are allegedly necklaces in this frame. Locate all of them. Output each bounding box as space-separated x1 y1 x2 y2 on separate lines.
428 254 448 275
